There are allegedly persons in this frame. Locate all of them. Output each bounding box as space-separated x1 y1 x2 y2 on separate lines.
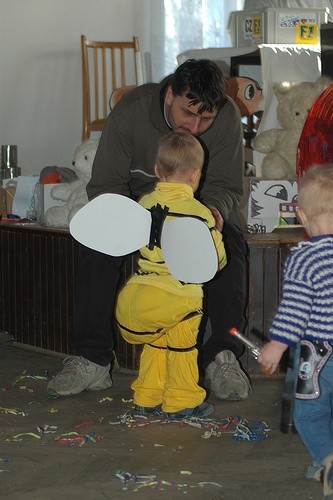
258 166 333 495
115 130 227 420
47 58 253 401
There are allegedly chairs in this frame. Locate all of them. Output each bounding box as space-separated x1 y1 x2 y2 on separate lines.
81 35 142 143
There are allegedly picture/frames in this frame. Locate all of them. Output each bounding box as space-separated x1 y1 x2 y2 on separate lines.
231 56 265 138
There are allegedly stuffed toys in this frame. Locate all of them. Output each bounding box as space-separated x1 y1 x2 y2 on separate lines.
45 138 101 228
251 76 333 181
226 77 263 118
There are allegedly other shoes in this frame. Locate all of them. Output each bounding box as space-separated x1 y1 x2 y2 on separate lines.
304 463 323 481
321 456 333 500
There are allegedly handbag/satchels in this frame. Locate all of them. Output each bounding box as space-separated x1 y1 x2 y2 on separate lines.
2 176 37 218
34 182 68 222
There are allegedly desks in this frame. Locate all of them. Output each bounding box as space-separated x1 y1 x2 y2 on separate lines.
0 219 309 378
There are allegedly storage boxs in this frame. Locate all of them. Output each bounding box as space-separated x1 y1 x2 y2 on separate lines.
227 7 322 48
234 177 306 233
0 176 70 227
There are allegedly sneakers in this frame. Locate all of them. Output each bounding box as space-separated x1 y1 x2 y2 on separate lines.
163 401 214 419
133 404 162 419
204 350 253 401
47 356 113 396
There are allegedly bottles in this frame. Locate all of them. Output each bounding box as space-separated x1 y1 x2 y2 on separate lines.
230 327 262 360
